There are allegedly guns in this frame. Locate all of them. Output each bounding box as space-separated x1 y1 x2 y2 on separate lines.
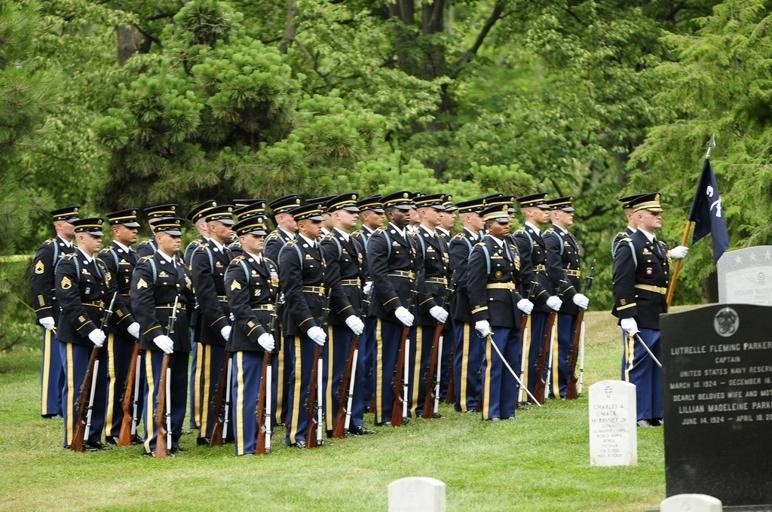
520 264 540 346
255 293 280 455
566 258 597 399
422 269 457 419
118 337 140 446
154 296 179 459
390 270 414 426
332 275 371 440
446 346 456 405
532 261 571 404
69 291 117 452
305 288 331 448
209 352 230 448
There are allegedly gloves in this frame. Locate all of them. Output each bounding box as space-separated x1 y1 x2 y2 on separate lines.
153 335 175 354
346 314 364 336
258 332 276 352
394 303 415 328
572 292 589 309
474 319 491 338
127 322 140 338
39 316 55 330
88 328 107 348
517 299 534 315
621 317 637 336
363 281 374 295
668 245 690 259
546 295 562 311
429 304 449 324
306 325 326 346
221 325 232 341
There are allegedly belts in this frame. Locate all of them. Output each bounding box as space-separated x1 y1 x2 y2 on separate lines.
251 304 275 312
388 269 416 280
532 264 546 272
81 299 105 308
424 276 449 288
562 267 582 279
486 280 516 293
154 302 186 311
50 288 55 299
302 286 325 296
634 284 667 295
217 296 228 303
341 276 361 289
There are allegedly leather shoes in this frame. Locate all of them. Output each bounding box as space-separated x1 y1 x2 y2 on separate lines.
197 437 210 445
289 413 441 448
64 434 143 452
637 417 664 427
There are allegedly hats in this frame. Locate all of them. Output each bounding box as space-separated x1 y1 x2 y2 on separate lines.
619 193 664 213
268 192 458 221
144 204 185 236
187 199 268 236
455 193 575 223
106 208 141 227
50 207 105 235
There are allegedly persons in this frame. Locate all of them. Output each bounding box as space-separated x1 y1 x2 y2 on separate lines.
30 205 146 451
263 190 379 450
128 201 197 456
351 192 457 428
609 192 672 428
609 191 690 267
185 199 283 455
448 191 590 423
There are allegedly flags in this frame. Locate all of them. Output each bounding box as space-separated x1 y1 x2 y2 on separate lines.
687 157 732 267
